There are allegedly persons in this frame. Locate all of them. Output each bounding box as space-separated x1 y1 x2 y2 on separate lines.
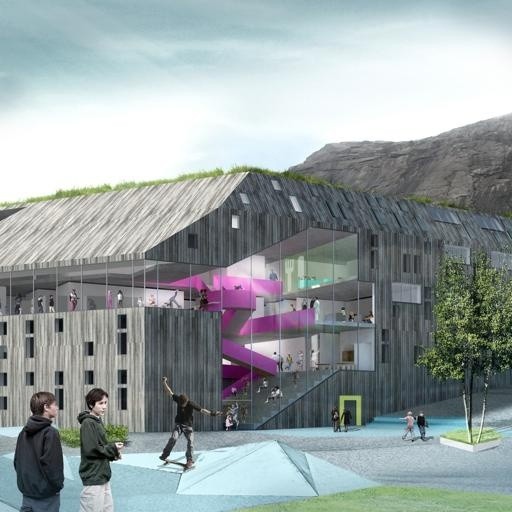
416 412 426 441
223 265 375 431
14 392 64 512
77 388 123 512
13 287 210 315
159 376 223 469
400 411 415 441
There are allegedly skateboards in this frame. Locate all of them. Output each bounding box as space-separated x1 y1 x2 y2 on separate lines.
159 457 195 471
